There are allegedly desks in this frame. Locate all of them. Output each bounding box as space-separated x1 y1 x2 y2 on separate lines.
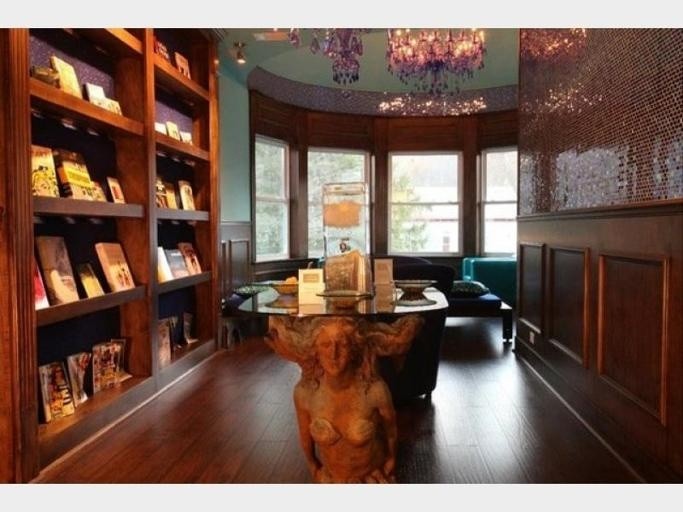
238 282 450 408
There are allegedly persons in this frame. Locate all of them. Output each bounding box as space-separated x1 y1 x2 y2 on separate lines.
264 311 425 483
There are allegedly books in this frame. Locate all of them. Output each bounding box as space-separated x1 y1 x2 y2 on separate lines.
156 176 196 210
158 242 202 283
31 55 122 115
29 144 126 202
156 120 194 144
33 236 135 310
38 338 131 423
158 312 199 363
157 42 192 79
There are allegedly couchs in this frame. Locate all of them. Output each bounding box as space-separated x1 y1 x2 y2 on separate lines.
395 248 455 300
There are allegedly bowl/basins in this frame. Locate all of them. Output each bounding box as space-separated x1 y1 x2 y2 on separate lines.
391 279 438 293
390 294 438 307
314 291 370 308
264 295 300 309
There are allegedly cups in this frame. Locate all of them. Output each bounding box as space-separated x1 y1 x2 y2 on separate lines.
373 281 397 313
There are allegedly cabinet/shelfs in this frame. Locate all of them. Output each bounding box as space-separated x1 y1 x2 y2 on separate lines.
2 30 220 484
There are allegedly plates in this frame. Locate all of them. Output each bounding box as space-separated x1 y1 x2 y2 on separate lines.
267 283 298 295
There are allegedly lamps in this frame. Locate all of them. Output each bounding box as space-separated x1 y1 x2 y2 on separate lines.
285 27 487 96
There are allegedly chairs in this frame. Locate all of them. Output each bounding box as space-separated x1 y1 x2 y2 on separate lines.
462 252 517 308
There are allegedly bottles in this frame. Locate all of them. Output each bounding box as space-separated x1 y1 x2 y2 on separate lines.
320 180 369 290
